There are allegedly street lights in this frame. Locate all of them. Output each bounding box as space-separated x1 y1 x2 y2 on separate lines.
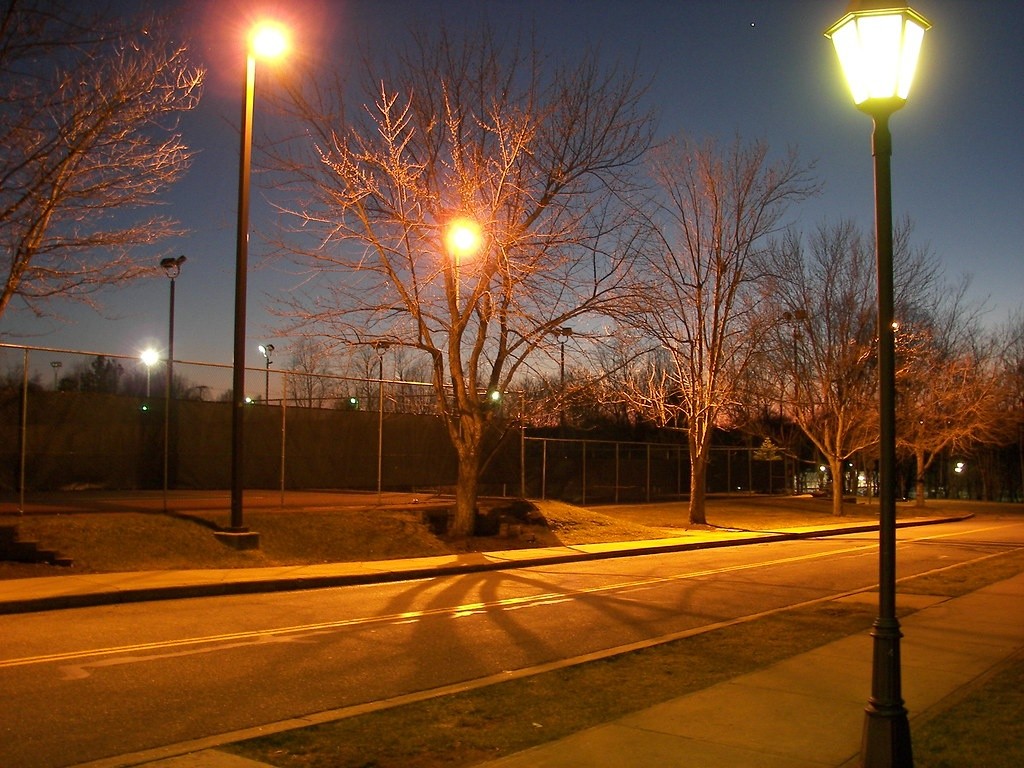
372 342 391 505
51 361 62 388
553 327 574 430
824 0 933 768
160 255 188 518
258 344 274 406
230 14 282 532
781 309 806 416
452 226 469 312
